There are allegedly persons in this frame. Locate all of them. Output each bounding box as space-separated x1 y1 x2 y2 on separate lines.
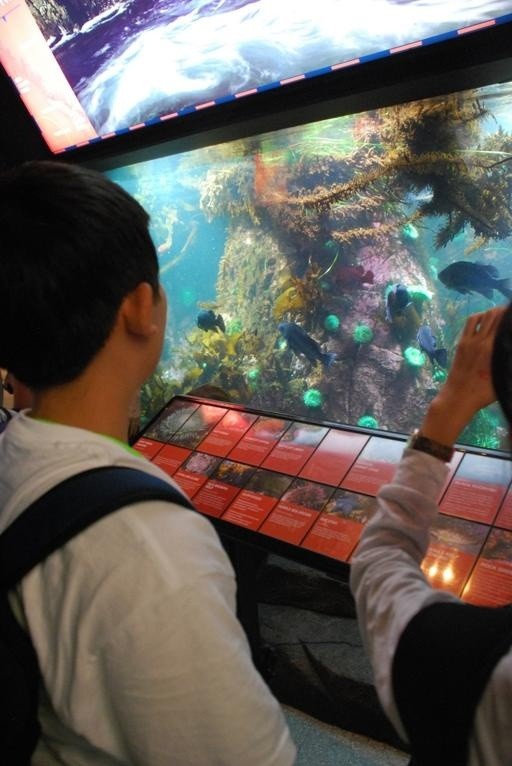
350 298 510 766
1 162 297 766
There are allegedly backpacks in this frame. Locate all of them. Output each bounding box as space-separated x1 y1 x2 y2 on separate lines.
0 463 206 765
